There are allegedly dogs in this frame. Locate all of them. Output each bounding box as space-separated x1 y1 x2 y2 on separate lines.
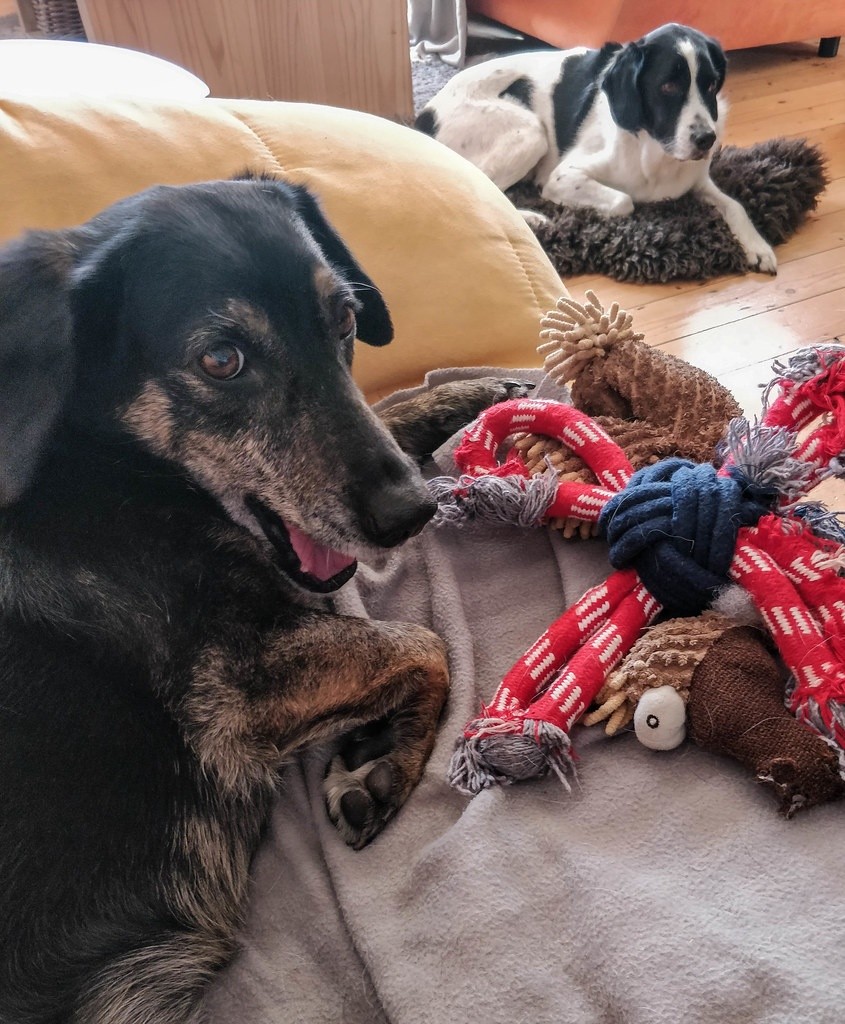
412 22 779 273
0 170 454 1024
